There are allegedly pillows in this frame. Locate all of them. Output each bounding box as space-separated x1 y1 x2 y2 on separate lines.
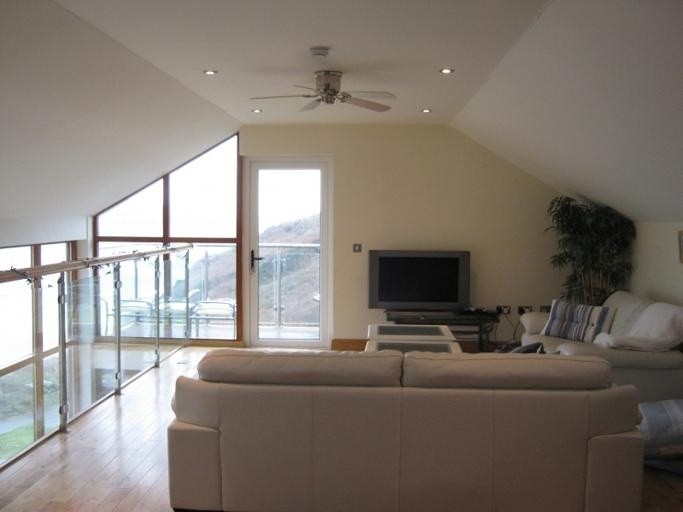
540 298 617 343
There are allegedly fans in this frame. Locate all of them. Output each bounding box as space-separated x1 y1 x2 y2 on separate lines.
250 70 396 112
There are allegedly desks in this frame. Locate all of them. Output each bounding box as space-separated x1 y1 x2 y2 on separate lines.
387 311 500 351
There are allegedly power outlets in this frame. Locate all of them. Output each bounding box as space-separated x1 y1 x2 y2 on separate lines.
519 306 532 314
497 305 510 314
540 306 550 312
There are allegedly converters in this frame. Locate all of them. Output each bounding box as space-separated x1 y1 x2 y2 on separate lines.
486 310 499 317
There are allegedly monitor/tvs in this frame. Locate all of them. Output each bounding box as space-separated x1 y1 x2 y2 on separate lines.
368 250 470 311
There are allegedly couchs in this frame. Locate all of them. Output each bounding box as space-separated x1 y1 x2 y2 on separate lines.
169 348 646 512
521 290 683 403
